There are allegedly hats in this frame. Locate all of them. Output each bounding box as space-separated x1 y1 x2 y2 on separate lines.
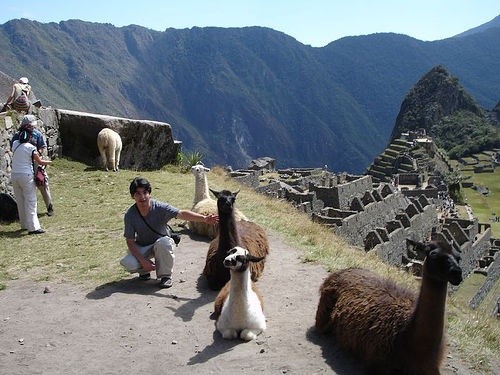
20 114 36 127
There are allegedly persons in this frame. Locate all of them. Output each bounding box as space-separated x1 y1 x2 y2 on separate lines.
119 177 219 288
10 115 53 216
12 128 53 235
4 77 32 111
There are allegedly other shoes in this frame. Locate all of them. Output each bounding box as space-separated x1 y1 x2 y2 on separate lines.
159 277 173 288
27 229 46 236
21 228 27 231
47 203 54 216
139 273 151 280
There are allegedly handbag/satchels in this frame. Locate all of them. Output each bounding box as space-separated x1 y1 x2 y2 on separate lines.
35 169 47 187
